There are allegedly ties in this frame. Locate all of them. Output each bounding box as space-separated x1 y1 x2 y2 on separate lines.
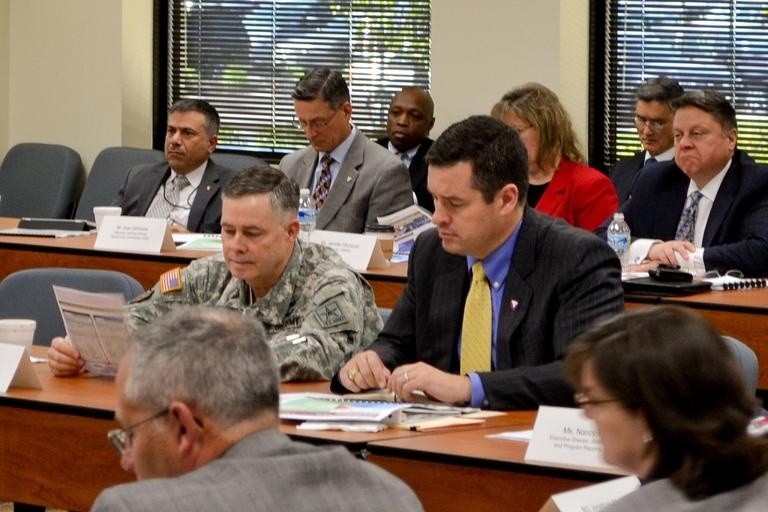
458 259 495 380
145 175 192 223
631 113 673 132
673 190 705 247
311 155 336 215
396 152 407 162
644 157 657 168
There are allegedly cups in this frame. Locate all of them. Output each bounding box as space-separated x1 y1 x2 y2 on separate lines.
93 206 121 236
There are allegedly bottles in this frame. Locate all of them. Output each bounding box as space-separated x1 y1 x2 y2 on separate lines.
297 189 315 243
606 214 629 276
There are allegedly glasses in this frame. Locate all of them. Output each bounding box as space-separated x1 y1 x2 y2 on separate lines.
573 388 624 416
290 110 339 130
106 397 198 460
512 123 533 139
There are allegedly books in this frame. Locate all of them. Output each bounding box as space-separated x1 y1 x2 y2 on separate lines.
174 232 225 255
278 387 510 431
697 273 766 291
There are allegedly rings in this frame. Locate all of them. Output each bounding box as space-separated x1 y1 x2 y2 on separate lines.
348 366 359 380
402 370 410 383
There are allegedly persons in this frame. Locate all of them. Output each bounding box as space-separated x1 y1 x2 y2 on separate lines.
275 64 416 236
86 302 426 511
490 81 621 234
363 85 441 218
110 98 240 235
603 73 683 212
567 302 767 511
328 112 625 415
587 89 766 279
46 164 386 386
